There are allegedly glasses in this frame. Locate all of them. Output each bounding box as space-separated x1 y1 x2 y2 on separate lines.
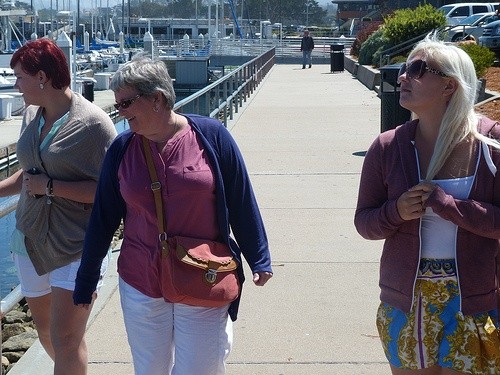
114 93 144 110
396 58 448 84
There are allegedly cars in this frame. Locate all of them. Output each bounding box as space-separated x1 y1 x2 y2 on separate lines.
437 3 500 50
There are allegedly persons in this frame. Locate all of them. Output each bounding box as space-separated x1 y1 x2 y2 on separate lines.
0 37 118 375
354 40 500 375
73 53 273 375
300 30 314 69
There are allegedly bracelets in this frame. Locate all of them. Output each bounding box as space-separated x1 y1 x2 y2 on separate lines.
46 178 54 197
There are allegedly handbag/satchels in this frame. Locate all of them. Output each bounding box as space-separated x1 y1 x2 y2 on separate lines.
158 231 240 308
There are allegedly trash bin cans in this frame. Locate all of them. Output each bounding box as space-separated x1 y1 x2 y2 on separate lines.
329 42 345 72
378 63 412 133
81 81 96 102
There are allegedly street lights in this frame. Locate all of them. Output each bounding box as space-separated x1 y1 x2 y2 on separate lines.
79 24 85 35
139 17 150 32
275 22 282 43
304 1 313 27
259 20 272 46
